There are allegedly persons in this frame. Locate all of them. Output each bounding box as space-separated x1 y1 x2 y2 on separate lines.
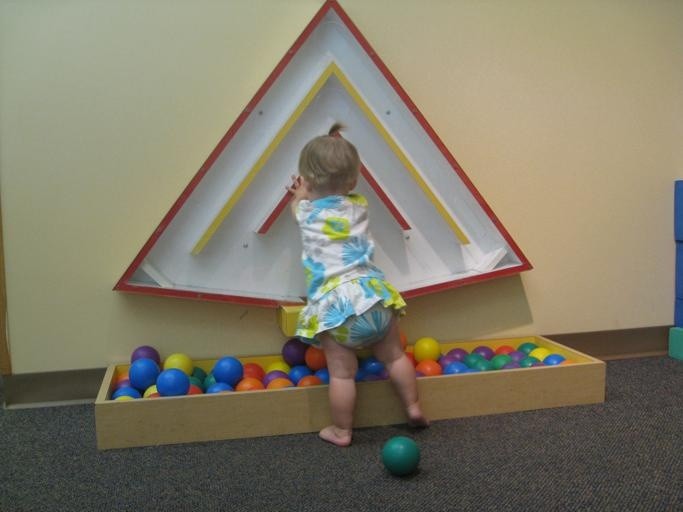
284 123 430 448
